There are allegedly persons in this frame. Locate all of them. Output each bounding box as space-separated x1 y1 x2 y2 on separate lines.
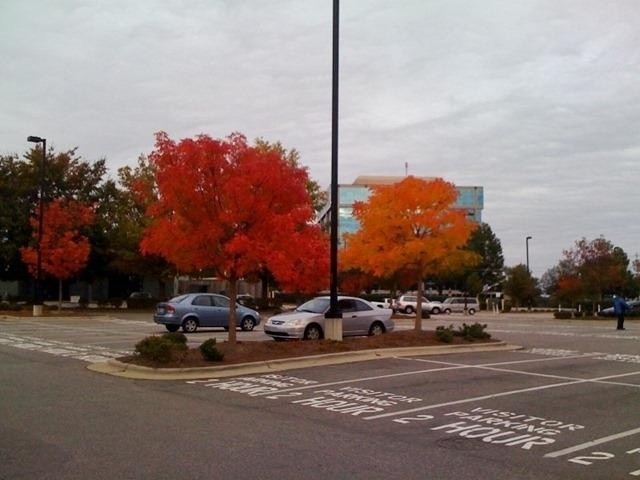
612 290 633 330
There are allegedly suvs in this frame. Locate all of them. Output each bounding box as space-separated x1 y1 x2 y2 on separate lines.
397 295 443 313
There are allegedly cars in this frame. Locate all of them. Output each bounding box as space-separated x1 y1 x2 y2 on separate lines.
263 295 395 344
599 300 640 318
152 293 261 334
122 287 152 309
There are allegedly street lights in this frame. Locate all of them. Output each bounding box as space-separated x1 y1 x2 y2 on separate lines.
27 135 48 302
526 236 533 272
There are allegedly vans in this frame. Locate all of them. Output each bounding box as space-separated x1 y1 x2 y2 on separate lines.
441 297 480 315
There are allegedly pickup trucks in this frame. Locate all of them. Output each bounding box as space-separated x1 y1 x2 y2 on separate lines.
371 297 398 312
